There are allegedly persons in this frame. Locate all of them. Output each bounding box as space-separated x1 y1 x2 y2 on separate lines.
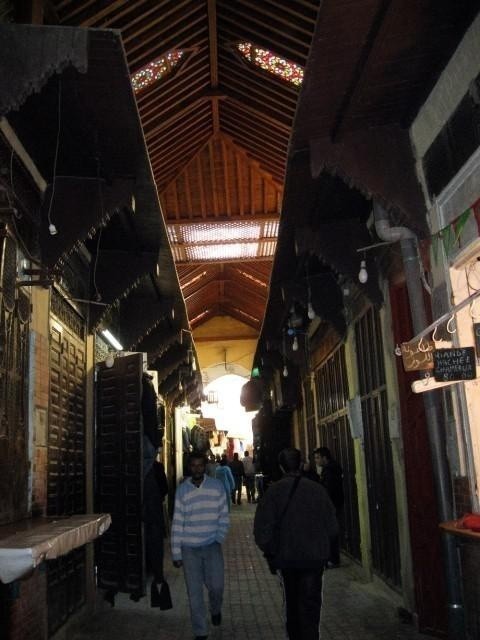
205 450 264 506
312 447 344 568
170 451 231 640
254 449 339 640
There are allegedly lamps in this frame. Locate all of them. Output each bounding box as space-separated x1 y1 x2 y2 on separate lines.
96 293 120 369
280 268 336 379
349 239 398 283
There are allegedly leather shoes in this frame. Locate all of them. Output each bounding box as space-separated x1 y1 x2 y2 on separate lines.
211 614 221 625
